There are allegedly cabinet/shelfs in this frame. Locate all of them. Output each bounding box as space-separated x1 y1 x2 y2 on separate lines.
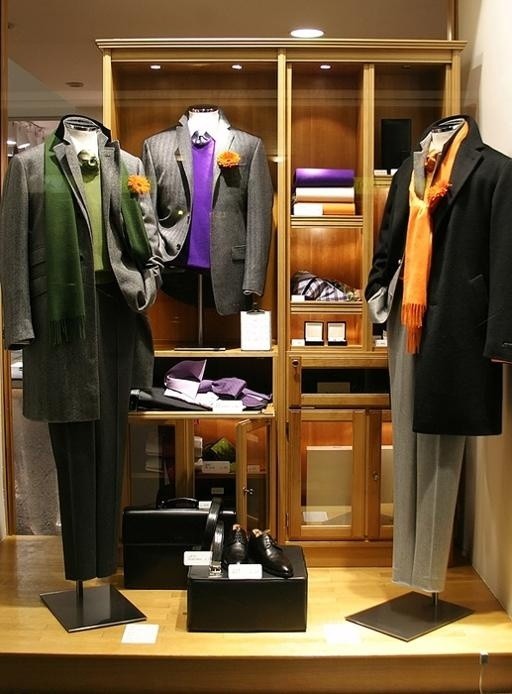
90 32 467 569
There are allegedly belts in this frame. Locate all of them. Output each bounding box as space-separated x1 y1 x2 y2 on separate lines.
200 497 225 562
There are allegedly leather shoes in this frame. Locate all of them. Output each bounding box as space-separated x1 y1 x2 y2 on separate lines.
221 523 248 571
249 528 293 577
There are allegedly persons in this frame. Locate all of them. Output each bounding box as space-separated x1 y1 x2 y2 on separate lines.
1 113 164 580
365 115 512 595
141 104 273 317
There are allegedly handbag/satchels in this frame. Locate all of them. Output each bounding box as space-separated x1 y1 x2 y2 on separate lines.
120 497 239 589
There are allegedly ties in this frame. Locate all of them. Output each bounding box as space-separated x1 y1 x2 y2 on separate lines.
292 267 362 302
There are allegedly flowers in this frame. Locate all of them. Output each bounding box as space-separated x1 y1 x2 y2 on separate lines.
427 179 452 206
215 151 240 169
127 174 150 195
